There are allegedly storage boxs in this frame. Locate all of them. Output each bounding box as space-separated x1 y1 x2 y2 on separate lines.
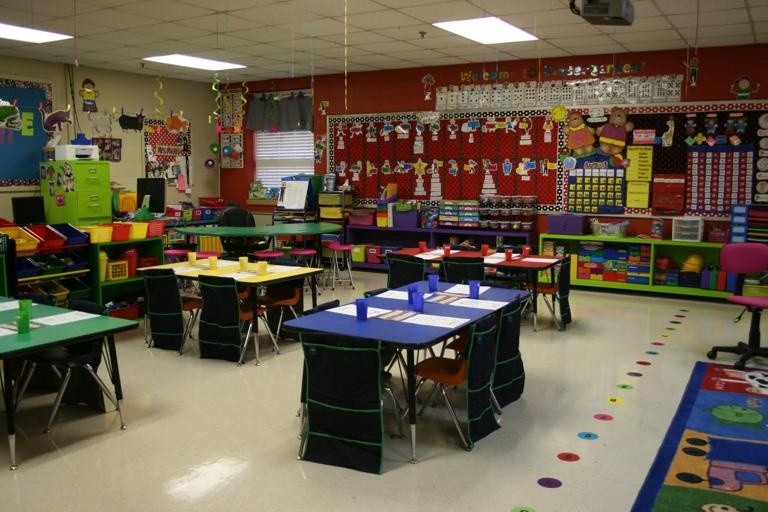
347 212 377 225
439 210 458 216
75 224 112 243
165 205 182 217
380 246 403 265
28 254 66 281
107 303 139 320
365 244 390 263
317 192 353 205
0 226 40 257
591 223 626 238
54 253 89 278
439 200 458 205
458 200 478 205
112 221 150 239
23 225 66 253
439 220 457 226
653 173 686 213
198 197 224 207
55 277 92 303
349 242 375 262
181 208 192 221
458 221 479 227
439 215 458 221
439 205 458 210
17 285 42 300
134 219 165 237
0 234 8 254
46 223 89 251
458 205 478 211
37 280 70 306
0 218 15 228
318 207 352 218
200 208 214 220
97 223 132 241
15 257 40 283
105 259 128 280
458 211 479 217
391 205 424 228
546 214 591 236
671 216 704 242
192 208 201 220
458 216 479 222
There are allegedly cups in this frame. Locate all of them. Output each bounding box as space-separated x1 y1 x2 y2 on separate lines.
505 249 512 262
470 280 480 299
481 245 488 256
412 292 424 312
356 299 368 320
444 247 450 255
427 275 439 292
188 252 196 265
257 262 267 276
408 284 418 304
522 245 530 257
419 242 425 252
15 310 29 334
239 257 248 271
20 299 32 317
208 256 217 270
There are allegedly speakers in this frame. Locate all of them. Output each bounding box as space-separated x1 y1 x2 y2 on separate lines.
570 0 635 26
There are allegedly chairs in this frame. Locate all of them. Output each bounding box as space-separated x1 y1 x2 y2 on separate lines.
217 253 256 334
384 253 436 290
390 309 503 452
195 251 221 259
11 299 118 434
363 287 436 404
11 292 63 403
256 257 306 351
296 330 406 475
441 256 485 285
520 254 572 331
295 299 339 417
705 241 767 370
443 293 525 411
197 274 280 365
218 207 272 263
437 245 481 284
141 269 202 355
483 246 527 289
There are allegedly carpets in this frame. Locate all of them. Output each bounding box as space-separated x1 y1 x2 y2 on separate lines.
630 361 767 512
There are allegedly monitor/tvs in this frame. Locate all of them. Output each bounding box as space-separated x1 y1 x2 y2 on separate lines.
11 196 45 226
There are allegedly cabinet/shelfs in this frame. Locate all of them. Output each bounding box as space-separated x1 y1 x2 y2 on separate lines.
537 232 767 304
0 233 96 308
90 236 165 315
38 159 112 227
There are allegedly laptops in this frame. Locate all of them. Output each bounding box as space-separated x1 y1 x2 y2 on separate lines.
136 178 165 213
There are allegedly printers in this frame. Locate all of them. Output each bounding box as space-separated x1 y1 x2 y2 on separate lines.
54 145 98 161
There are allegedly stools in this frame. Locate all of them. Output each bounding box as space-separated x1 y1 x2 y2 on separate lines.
315 240 341 284
163 250 192 263
288 250 320 295
325 243 355 290
254 251 284 265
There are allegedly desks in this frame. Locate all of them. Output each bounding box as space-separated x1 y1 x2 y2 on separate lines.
283 280 531 464
135 259 324 364
0 296 139 471
376 248 566 332
169 222 342 249
